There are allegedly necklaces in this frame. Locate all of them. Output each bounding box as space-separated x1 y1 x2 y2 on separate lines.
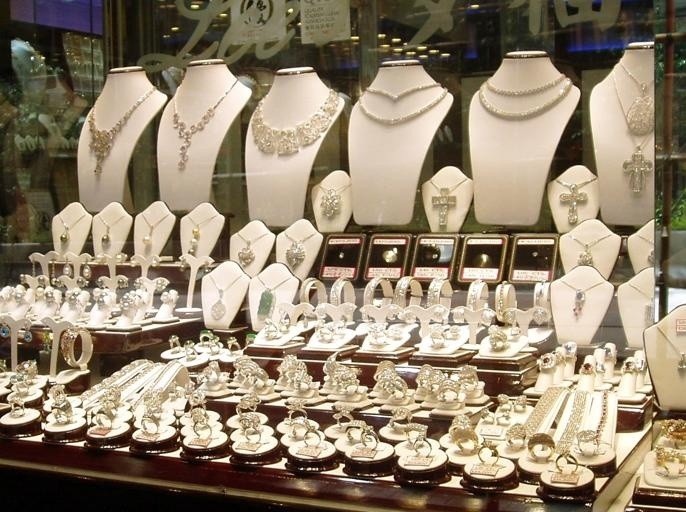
58 60 655 334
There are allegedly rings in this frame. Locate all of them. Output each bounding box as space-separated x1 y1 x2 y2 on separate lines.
1 320 685 485
